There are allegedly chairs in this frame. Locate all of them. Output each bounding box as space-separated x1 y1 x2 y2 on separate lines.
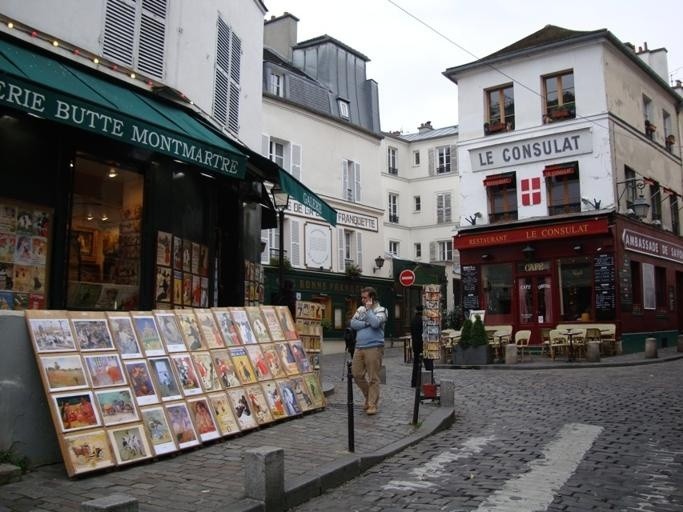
541 327 604 361
443 330 535 364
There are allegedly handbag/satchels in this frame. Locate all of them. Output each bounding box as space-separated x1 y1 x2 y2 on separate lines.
344 326 355 357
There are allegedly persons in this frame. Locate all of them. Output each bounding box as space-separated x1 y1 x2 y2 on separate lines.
156 244 209 307
350 287 388 416
409 304 435 387
59 308 310 435
1 206 47 309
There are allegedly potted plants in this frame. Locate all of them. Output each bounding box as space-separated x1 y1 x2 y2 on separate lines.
489 118 506 132
550 102 575 117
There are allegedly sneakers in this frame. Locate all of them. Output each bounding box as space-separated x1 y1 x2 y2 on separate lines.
363 400 376 414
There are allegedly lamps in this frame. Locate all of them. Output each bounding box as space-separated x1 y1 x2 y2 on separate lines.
615 178 651 218
